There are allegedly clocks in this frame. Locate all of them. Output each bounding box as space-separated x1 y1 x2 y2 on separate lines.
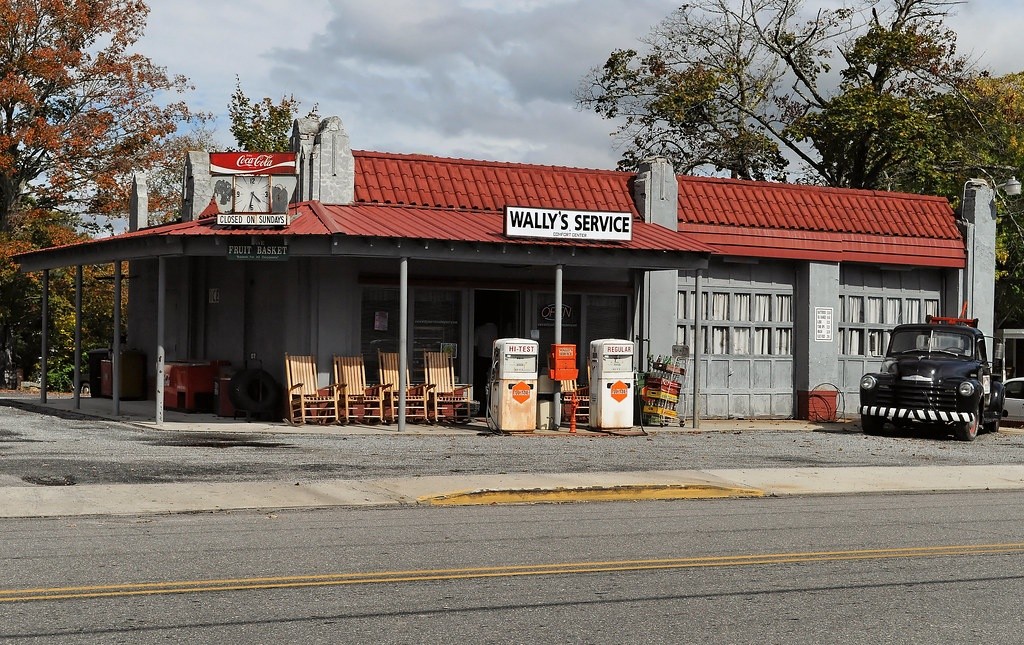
231 174 274 214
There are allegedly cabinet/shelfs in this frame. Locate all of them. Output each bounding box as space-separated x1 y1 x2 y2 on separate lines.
641 352 691 427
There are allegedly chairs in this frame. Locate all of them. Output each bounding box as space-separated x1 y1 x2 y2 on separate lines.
421 348 474 425
375 348 432 426
331 352 388 425
283 351 344 427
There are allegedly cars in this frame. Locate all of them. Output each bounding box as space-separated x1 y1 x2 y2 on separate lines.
1001 377 1024 423
860 324 1006 442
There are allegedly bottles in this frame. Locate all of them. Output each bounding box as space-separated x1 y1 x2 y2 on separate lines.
645 355 689 413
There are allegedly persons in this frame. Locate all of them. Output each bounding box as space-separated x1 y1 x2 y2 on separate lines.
474 314 499 364
